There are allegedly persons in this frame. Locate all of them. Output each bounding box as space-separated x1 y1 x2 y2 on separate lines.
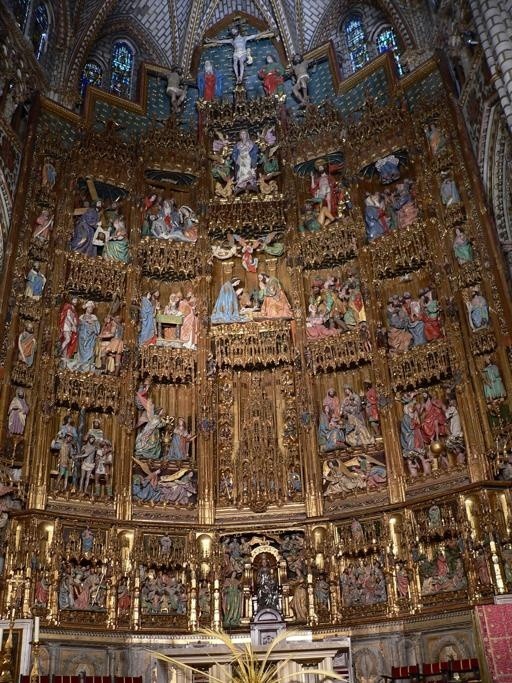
160 27 315 113
400 391 465 478
386 287 443 353
18 324 37 370
30 500 509 620
316 378 387 501
452 225 472 265
296 123 462 239
32 156 199 263
216 370 301 503
211 230 294 323
231 129 263 198
130 380 196 506
481 354 507 403
7 386 29 436
59 286 198 375
24 261 46 302
50 416 113 500
467 286 488 332
305 263 372 351
